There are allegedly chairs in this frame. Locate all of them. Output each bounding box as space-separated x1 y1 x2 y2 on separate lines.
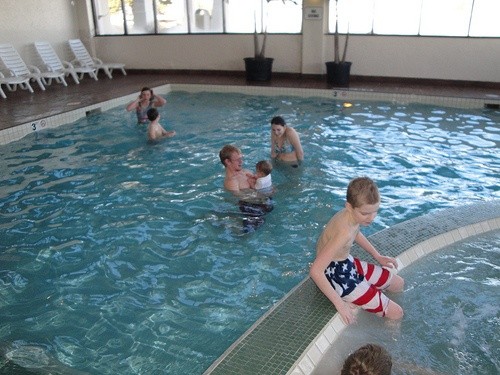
0 39 128 98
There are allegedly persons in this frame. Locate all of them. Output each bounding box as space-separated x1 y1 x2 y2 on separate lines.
147 108 174 139
341 343 392 375
219 145 253 190
128 87 167 123
247 160 272 190
309 177 405 323
270 118 303 159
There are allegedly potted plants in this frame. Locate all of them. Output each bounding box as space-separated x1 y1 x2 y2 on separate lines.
239 0 297 83
322 0 352 86
